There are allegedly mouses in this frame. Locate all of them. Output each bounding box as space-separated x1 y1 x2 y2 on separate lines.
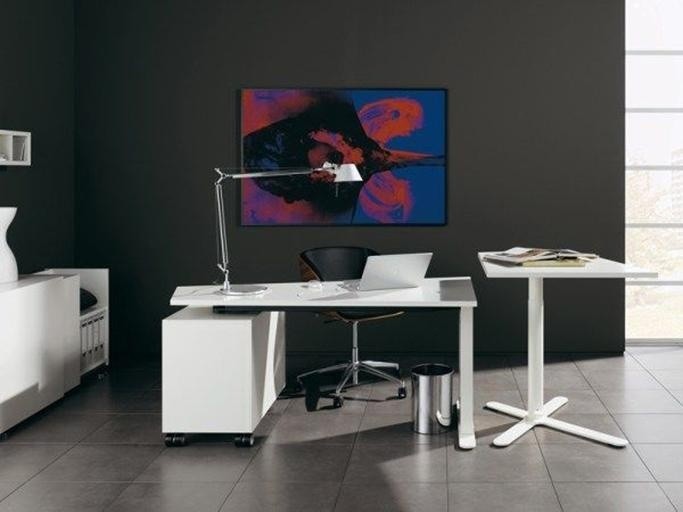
309 280 322 289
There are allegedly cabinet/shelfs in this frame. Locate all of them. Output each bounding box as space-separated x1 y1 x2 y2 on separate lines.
159 305 286 435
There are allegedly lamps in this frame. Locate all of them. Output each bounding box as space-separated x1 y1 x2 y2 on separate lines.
212 161 364 296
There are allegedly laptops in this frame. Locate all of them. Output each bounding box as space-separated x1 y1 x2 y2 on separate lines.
341 252 433 291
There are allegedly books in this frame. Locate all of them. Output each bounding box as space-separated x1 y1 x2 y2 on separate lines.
485 245 596 265
521 259 585 268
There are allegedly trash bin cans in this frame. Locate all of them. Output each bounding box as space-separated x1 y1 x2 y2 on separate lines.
409 363 456 435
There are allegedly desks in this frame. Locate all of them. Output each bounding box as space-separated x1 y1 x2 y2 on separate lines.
167 274 480 451
476 247 658 449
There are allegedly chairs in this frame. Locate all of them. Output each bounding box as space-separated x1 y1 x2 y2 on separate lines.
295 246 409 408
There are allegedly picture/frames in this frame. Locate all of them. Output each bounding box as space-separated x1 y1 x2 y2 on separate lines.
234 86 449 227
